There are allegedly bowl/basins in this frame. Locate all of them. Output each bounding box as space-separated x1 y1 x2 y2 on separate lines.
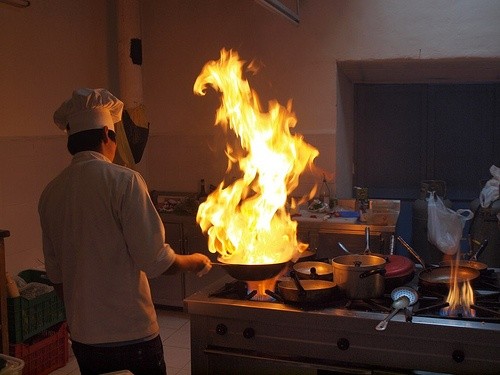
292 247 319 262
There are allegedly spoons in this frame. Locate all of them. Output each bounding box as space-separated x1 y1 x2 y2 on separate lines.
375 296 410 330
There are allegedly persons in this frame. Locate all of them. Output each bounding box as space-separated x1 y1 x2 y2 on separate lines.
38 88 213 375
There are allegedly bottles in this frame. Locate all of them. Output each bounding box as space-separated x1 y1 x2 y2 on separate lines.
319 180 330 209
196 179 208 204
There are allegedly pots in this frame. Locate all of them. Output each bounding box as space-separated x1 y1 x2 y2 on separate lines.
332 254 386 301
209 255 291 280
277 270 336 302
293 260 332 278
371 232 415 286
397 234 480 288
438 240 489 271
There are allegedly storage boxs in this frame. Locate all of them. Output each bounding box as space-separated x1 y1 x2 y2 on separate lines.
0 272 68 375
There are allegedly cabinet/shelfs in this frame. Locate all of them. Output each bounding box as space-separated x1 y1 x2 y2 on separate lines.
147 216 355 311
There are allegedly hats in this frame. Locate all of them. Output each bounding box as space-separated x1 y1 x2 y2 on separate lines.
54 89 125 134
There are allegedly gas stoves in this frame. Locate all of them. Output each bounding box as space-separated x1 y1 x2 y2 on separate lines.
184 264 500 375
